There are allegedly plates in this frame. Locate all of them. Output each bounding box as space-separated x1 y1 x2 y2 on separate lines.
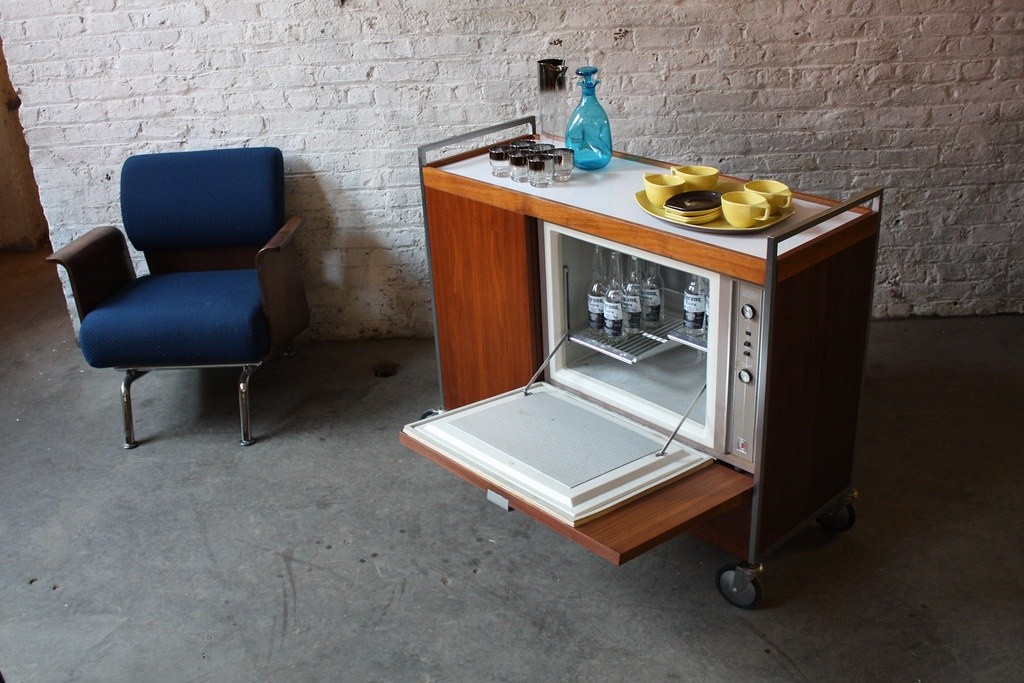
663 191 722 224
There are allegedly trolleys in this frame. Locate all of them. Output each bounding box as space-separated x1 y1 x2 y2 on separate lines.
399 116 884 610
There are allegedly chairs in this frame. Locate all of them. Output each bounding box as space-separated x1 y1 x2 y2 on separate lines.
44 146 306 449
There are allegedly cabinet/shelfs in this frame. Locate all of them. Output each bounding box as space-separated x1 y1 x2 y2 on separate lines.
400 116 886 610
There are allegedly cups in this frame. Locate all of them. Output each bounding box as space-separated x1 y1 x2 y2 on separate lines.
721 191 770 227
643 171 686 209
489 139 574 187
744 179 792 215
671 165 719 191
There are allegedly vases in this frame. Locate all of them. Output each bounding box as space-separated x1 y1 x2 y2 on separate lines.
566 67 615 169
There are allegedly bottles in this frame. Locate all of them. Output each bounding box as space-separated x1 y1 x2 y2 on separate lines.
588 244 665 341
703 289 709 339
565 66 612 170
683 274 707 335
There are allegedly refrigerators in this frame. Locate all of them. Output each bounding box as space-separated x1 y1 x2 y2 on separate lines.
403 222 763 529
